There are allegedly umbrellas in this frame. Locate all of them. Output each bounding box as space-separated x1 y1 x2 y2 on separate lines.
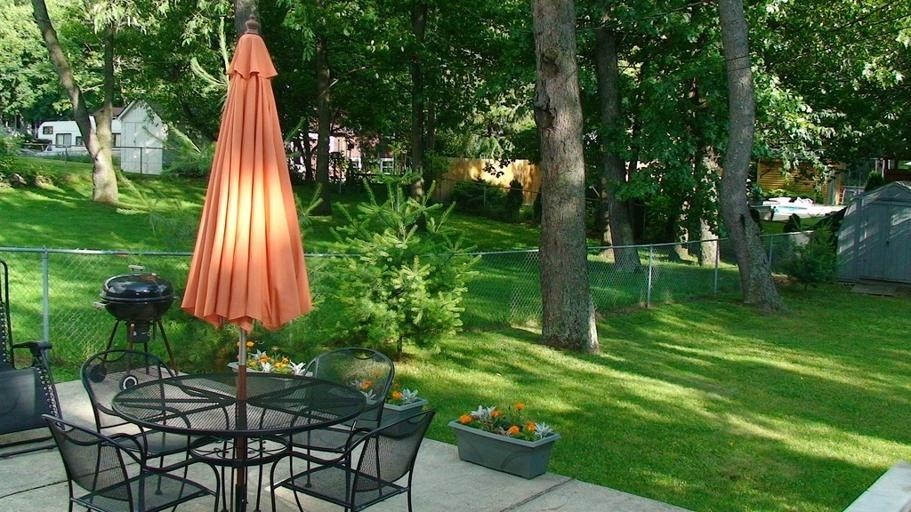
179 15 316 512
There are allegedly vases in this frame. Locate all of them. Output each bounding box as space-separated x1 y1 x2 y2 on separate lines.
227 359 303 402
341 393 428 432
447 413 554 478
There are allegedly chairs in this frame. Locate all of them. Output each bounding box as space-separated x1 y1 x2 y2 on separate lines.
81 346 232 512
267 406 436 512
40 411 224 512
0 257 67 458
250 346 395 512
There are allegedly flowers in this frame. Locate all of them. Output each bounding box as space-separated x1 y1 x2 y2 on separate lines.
460 402 557 446
237 336 307 379
344 374 421 407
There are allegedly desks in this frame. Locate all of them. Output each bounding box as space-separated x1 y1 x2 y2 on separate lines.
109 370 365 512
